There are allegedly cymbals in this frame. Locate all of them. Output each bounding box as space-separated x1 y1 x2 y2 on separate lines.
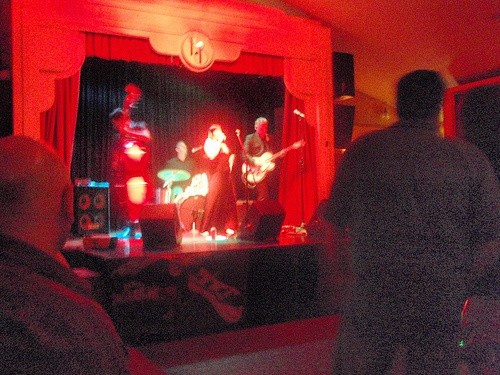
157 168 191 181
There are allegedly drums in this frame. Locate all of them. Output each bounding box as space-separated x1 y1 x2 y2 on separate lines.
174 192 206 232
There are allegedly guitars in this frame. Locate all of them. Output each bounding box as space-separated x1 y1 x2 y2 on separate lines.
242 139 305 183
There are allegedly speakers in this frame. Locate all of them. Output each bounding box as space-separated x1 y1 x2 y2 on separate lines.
304 200 329 236
139 203 182 251
237 199 286 243
72 182 110 236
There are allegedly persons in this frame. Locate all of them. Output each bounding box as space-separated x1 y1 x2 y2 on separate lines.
242 117 288 201
200 124 238 239
323 70 500 375
0 134 129 375
166 141 195 202
109 109 150 240
